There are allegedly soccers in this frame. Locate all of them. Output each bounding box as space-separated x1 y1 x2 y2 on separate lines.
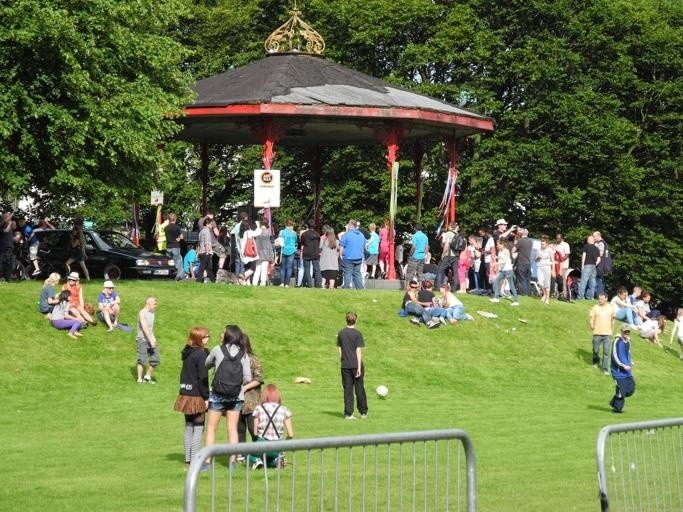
375 385 390 398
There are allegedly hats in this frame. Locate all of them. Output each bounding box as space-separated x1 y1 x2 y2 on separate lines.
102 281 115 287
494 219 508 226
621 323 631 331
67 272 80 280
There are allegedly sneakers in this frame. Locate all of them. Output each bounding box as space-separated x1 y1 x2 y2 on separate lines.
32 269 41 275
410 318 457 329
202 462 210 470
106 324 122 333
136 376 156 384
232 455 286 469
489 292 520 307
368 272 386 279
541 295 549 304
68 321 97 339
465 313 475 321
345 413 368 419
632 324 641 330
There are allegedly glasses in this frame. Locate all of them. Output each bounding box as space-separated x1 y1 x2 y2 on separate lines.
69 279 75 281
410 285 418 289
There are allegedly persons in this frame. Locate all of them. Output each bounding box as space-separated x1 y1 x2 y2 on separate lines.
401 307 468 330
178 320 215 480
153 208 396 291
610 287 660 329
0 203 58 282
251 380 295 473
66 226 93 283
668 307 683 360
395 220 612 307
203 324 253 474
237 333 264 462
589 291 616 377
38 273 120 332
41 306 122 341
610 306 668 350
609 323 640 416
131 297 164 382
339 311 372 420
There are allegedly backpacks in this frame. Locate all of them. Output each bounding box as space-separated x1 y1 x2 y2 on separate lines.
448 230 466 251
212 345 245 396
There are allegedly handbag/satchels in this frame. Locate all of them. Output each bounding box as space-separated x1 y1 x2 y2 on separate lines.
243 238 257 257
275 237 284 249
599 240 612 275
85 303 95 314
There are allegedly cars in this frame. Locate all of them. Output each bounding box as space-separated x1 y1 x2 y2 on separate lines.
32 230 177 281
